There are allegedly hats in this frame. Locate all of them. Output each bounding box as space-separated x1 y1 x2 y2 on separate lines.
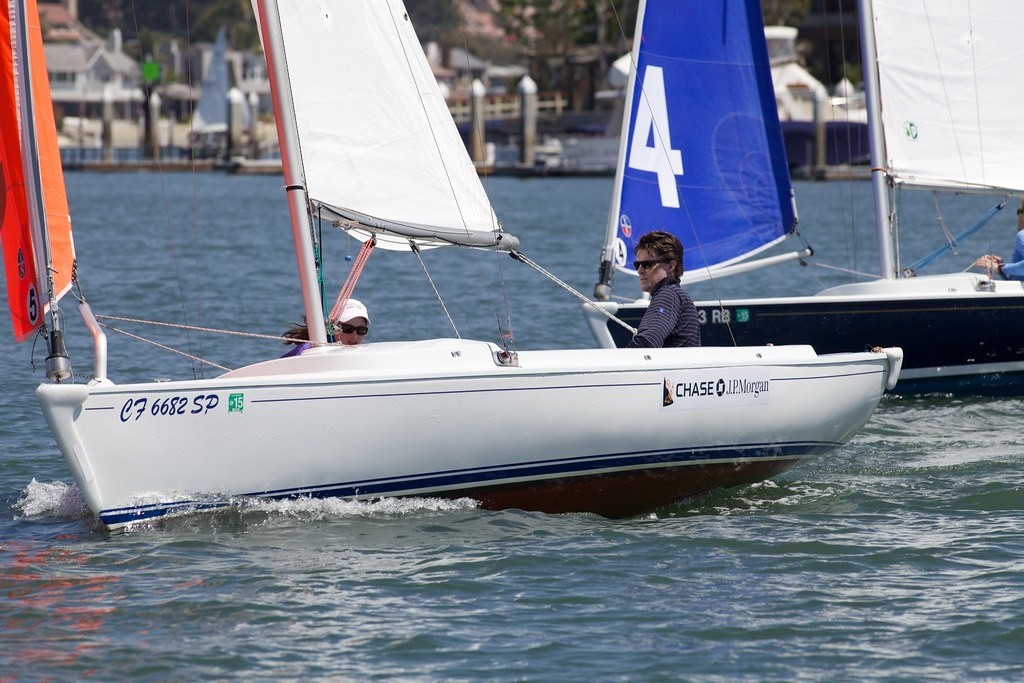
337 299 372 326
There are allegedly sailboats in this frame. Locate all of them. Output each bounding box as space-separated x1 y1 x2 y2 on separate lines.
0 0 905 520
584 0 1023 401
186 25 257 161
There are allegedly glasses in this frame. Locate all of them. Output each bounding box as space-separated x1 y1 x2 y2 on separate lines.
340 323 368 335
1017 208 1024 215
633 259 670 270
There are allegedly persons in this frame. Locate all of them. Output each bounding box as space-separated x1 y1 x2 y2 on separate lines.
280 298 370 359
628 231 703 348
977 195 1024 281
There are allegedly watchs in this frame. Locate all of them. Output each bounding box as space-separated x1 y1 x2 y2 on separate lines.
998 262 1004 274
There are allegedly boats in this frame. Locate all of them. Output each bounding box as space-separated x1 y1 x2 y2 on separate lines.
554 24 871 175
